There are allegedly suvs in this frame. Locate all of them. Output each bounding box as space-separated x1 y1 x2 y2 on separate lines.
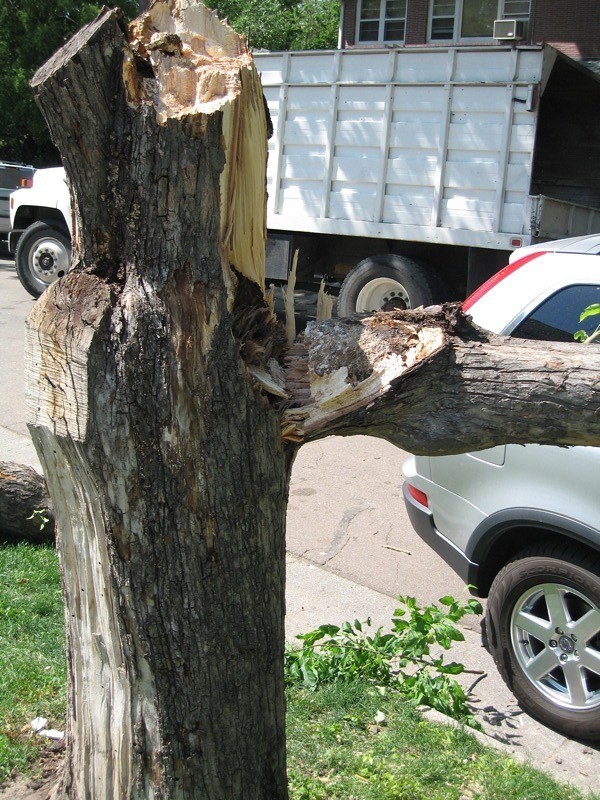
0 160 36 231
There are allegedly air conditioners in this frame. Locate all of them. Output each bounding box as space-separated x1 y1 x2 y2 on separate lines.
493 20 523 41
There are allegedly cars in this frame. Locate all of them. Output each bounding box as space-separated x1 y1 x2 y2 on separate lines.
400 232 600 748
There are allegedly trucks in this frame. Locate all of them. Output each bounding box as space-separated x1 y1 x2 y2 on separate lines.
5 43 599 322
